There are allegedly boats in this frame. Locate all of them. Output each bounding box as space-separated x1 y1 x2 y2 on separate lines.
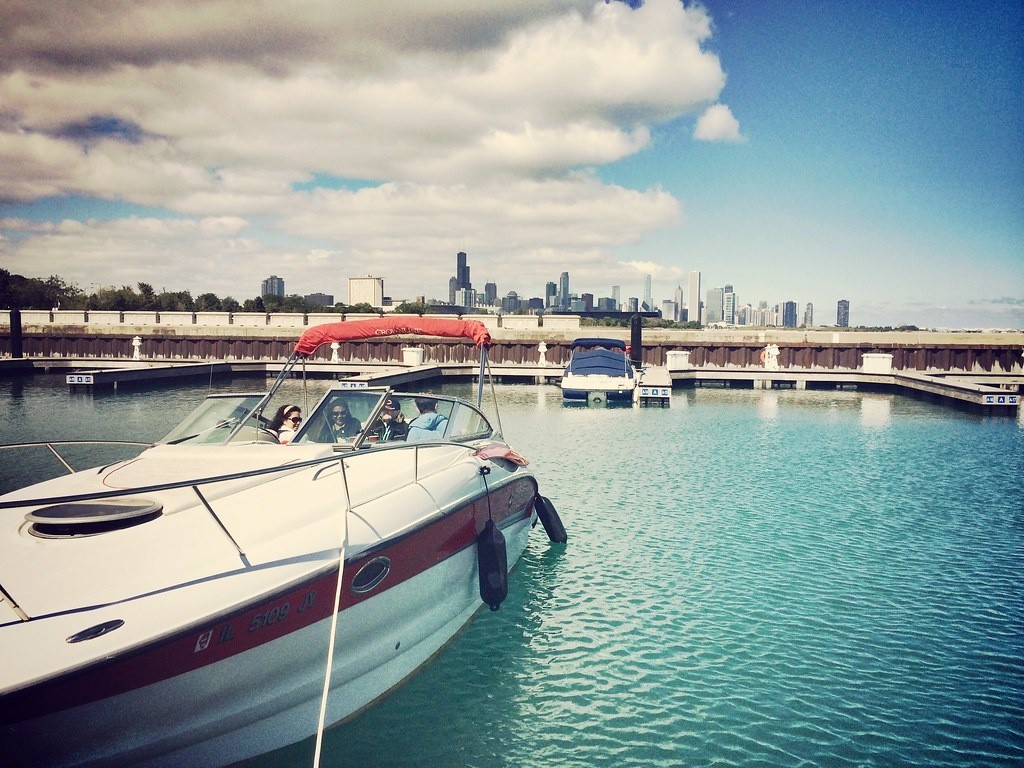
561 338 635 408
0 319 568 768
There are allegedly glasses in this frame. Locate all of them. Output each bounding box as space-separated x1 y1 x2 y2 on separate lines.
286 417 303 424
331 409 348 418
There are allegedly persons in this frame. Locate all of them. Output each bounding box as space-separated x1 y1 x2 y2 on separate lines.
367 398 408 441
269 405 308 444
406 395 449 440
317 397 361 443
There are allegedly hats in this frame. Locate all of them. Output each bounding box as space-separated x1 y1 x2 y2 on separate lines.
383 399 401 411
